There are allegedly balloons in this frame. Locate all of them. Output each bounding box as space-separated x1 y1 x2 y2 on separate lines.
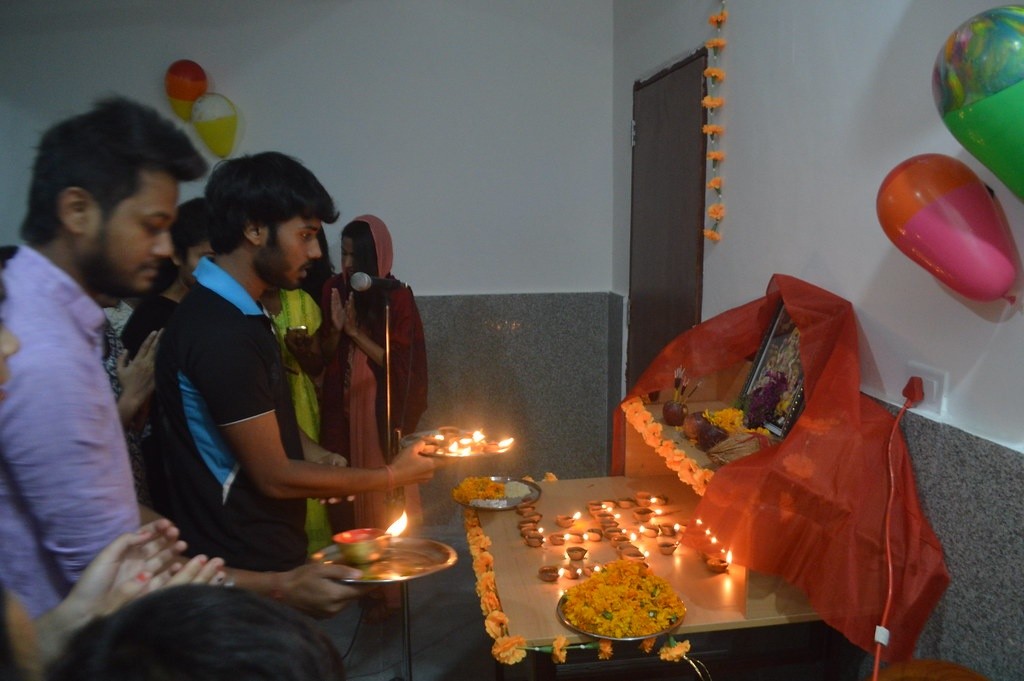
931 3 1024 203
165 61 239 160
875 152 1021 308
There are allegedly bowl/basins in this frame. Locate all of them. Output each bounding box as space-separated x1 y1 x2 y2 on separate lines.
333 528 390 561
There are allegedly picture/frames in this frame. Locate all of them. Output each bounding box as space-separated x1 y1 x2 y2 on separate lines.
736 292 848 439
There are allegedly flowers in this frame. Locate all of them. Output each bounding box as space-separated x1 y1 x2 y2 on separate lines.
461 506 508 639
523 475 534 482
491 629 691 662
701 1 729 242
541 472 559 482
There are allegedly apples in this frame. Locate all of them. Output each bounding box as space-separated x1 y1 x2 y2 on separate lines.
684 411 710 440
663 400 688 426
700 425 727 451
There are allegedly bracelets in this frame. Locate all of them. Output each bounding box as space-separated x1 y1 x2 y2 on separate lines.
379 462 394 497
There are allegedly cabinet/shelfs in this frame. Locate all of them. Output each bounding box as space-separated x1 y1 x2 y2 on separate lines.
625 351 888 619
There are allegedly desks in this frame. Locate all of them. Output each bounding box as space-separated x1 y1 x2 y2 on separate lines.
476 474 867 681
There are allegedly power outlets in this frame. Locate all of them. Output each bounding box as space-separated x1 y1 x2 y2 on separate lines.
901 358 952 417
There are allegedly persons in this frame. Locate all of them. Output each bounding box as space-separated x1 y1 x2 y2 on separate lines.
0 100 366 631
0 267 237 681
95 198 431 572
42 583 347 681
146 152 453 575
